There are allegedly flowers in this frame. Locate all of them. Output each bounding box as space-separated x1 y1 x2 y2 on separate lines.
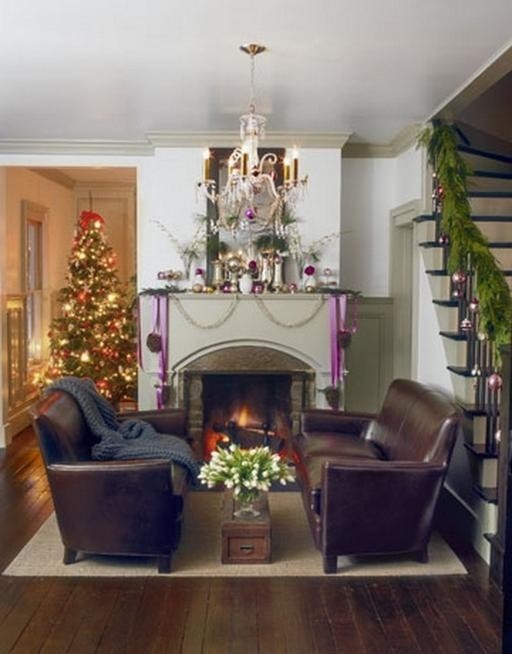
198 440 297 507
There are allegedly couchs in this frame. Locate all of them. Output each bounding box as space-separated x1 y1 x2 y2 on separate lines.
27 377 191 573
292 378 463 574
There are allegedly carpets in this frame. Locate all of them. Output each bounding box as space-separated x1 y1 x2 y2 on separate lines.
1 489 471 579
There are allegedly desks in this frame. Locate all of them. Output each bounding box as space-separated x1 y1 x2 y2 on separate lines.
221 488 273 564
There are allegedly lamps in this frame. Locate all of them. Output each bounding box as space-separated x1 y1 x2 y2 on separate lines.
194 43 308 240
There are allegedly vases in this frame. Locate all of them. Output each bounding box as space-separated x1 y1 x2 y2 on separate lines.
233 488 262 519
